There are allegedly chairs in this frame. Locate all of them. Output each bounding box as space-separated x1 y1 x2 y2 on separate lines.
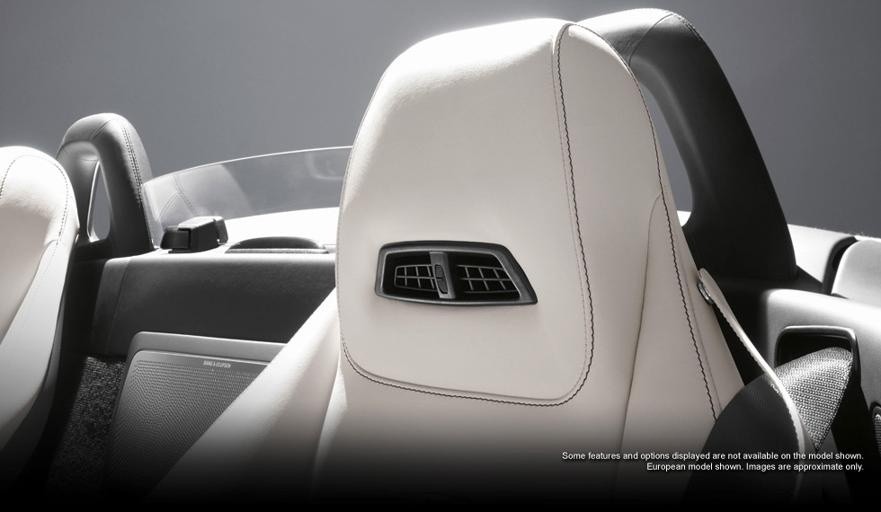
150 6 879 511
2 111 165 505
